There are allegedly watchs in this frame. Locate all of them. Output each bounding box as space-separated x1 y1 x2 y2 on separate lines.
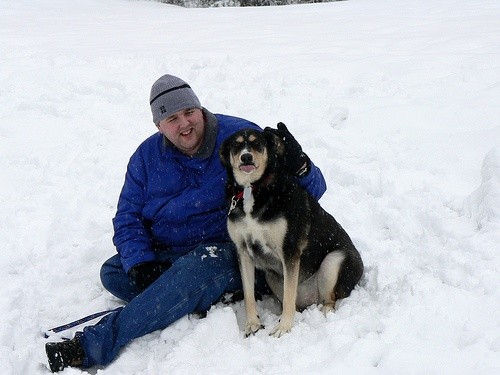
295 155 311 177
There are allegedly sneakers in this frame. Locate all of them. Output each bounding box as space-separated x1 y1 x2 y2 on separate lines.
46 333 84 373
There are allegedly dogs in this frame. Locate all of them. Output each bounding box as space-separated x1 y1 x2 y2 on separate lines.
218 128 365 339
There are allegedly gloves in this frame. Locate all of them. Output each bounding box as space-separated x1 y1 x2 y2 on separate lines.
129 261 163 294
264 123 311 177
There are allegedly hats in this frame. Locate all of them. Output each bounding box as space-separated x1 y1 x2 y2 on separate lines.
149 74 202 123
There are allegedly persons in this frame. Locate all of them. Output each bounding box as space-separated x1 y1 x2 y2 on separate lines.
44 74 327 373
